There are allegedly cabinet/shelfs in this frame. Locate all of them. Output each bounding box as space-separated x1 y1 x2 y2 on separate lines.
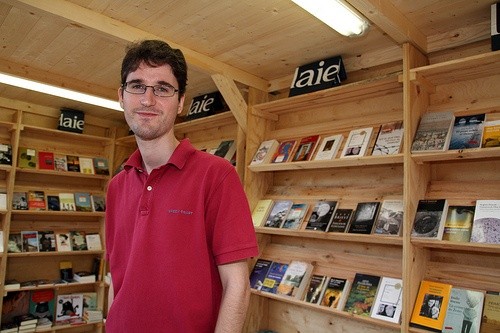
0 41 500 333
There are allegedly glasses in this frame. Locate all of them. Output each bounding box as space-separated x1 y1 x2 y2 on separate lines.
121 82 180 97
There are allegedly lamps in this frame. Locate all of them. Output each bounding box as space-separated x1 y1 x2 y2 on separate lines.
291 0 370 38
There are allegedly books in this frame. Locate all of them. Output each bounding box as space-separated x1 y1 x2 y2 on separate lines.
0 257 103 333
201 140 236 166
250 119 404 165
409 105 500 154
409 198 500 246
409 280 500 333
0 143 110 176
249 258 402 326
252 198 403 237
0 230 103 254
0 189 107 212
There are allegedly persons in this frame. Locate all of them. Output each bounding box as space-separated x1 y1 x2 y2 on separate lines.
420 294 441 320
104 39 259 333
